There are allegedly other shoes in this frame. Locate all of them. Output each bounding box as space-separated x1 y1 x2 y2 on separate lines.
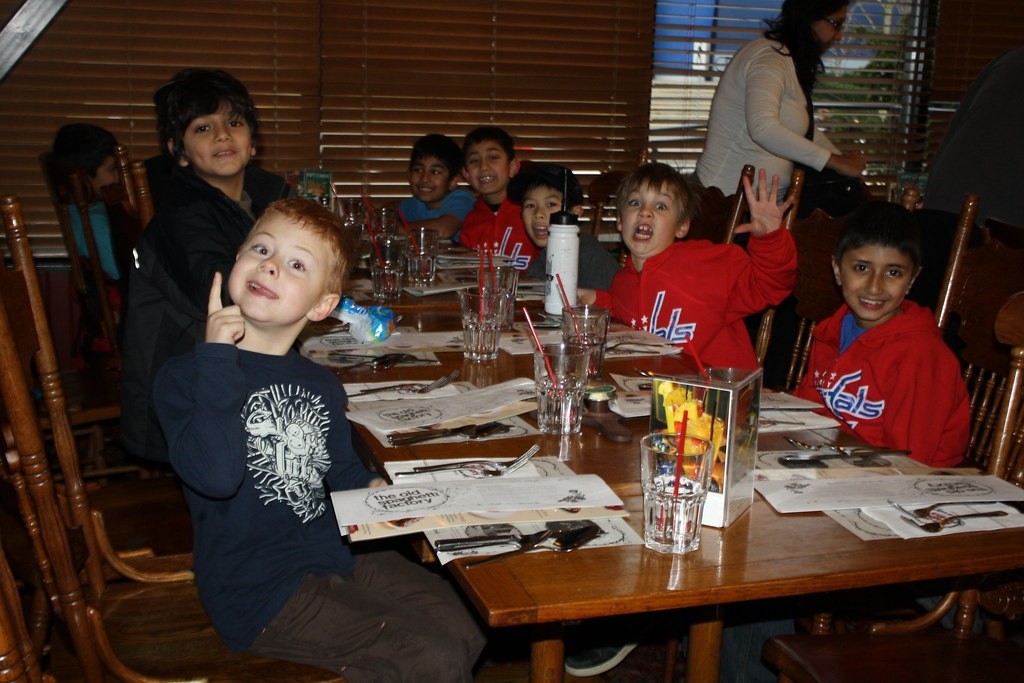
564 640 637 676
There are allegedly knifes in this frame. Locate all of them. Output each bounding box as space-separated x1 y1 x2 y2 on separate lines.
434 529 606 546
782 450 911 461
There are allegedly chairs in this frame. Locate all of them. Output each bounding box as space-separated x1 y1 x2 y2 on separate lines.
0 145 1024 683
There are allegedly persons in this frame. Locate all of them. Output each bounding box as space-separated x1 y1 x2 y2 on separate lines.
395 133 474 242
721 200 971 682
52 124 136 308
564 163 798 676
507 160 620 293
155 197 486 682
918 45 1024 311
459 125 541 269
696 0 865 248
117 67 299 476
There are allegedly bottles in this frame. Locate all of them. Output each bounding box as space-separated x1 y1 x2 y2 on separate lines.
545 168 580 315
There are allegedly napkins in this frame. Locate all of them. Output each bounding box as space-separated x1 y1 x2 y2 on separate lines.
605 342 683 360
342 380 461 403
609 372 654 392
859 503 1024 540
382 457 540 484
756 410 841 433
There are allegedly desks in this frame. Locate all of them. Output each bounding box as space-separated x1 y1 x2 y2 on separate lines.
333 237 549 310
295 309 1024 683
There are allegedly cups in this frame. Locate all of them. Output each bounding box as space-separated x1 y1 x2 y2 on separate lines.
369 237 408 302
365 208 399 240
405 229 439 289
330 196 362 249
640 433 714 554
561 305 610 381
534 344 592 435
477 267 518 333
460 287 507 361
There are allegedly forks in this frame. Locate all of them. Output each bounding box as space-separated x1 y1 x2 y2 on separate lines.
395 443 540 476
887 500 997 517
347 370 461 398
901 511 1009 533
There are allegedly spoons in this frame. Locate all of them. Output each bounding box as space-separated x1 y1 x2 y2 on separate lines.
438 530 553 552
789 438 890 452
464 526 601 569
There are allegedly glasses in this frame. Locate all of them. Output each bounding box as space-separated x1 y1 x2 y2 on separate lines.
821 16 844 32
